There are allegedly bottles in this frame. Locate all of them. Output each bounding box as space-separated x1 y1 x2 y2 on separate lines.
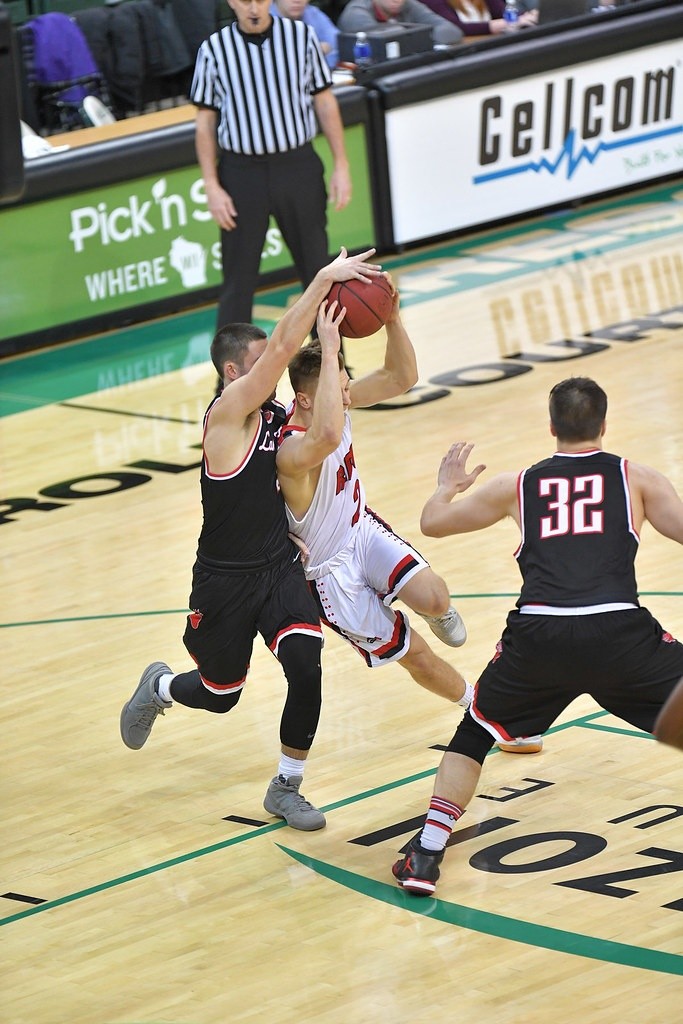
503 0 519 35
353 32 372 65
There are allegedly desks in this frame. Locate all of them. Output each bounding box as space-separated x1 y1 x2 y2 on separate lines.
0 0 683 360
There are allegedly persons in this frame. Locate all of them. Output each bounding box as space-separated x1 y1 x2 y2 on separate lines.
118 247 542 832
391 378 682 897
270 0 541 73
189 1 351 395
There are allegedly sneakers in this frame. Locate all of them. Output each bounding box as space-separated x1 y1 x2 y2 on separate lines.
263 773 326 831
391 826 447 895
414 604 467 647
495 734 544 753
119 661 173 751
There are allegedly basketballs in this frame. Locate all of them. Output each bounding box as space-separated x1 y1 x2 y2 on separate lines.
323 276 394 340
